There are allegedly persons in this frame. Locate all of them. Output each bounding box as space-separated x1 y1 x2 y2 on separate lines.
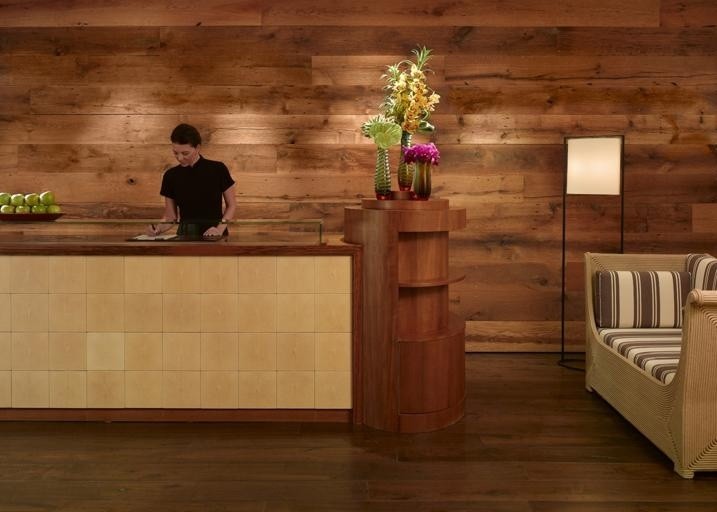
148 123 236 237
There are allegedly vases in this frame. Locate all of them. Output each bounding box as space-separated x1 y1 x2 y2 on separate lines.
415 160 432 200
373 148 392 197
397 124 414 192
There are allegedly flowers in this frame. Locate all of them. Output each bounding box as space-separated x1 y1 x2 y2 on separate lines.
354 40 443 165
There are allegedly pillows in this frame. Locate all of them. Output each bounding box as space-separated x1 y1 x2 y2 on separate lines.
594 270 692 329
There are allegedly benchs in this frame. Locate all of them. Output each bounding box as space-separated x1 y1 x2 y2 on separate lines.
573 245 717 482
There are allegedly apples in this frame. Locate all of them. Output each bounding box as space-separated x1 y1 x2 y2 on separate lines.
0 191 60 214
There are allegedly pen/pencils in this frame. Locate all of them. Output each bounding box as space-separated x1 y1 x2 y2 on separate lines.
152 224 155 230
